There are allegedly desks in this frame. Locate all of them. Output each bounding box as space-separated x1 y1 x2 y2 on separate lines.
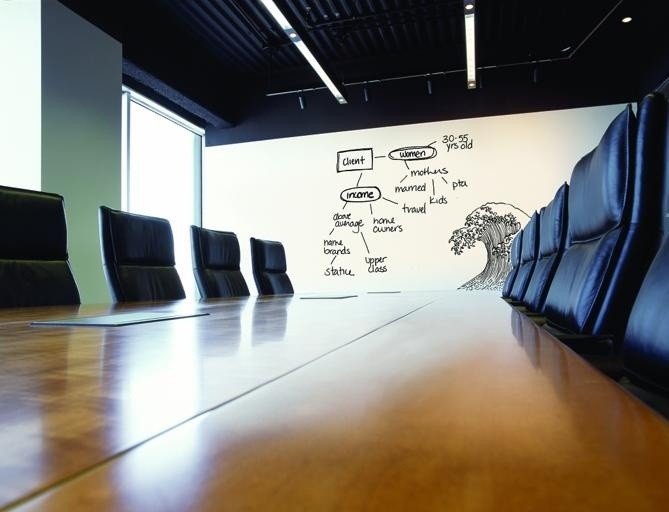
1 291 669 512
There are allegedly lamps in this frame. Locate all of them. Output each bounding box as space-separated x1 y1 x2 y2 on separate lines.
261 0 349 105
463 0 477 91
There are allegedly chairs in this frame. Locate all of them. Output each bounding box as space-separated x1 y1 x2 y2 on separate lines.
513 181 569 314
501 229 523 298
529 102 637 349
190 225 250 298
506 209 539 304
0 186 81 310
555 77 668 419
98 206 186 303
250 237 294 294
